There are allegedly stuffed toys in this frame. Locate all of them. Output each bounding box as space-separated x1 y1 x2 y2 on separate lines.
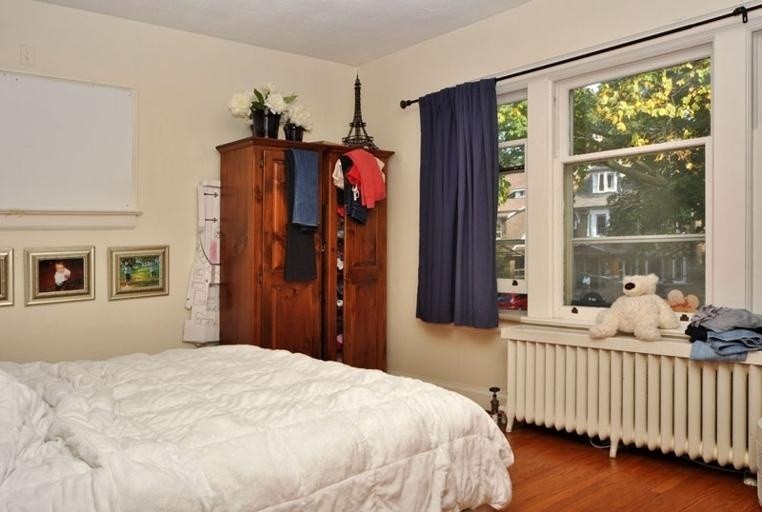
588 273 680 342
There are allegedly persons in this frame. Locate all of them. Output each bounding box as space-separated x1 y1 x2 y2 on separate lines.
121 260 133 288
54 261 72 290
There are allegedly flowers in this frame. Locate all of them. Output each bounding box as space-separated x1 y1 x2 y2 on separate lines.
228 83 298 125
281 104 312 133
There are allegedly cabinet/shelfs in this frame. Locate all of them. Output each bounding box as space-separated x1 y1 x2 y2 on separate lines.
216 136 396 372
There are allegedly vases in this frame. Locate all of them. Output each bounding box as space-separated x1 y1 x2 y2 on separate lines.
251 110 281 140
282 123 303 142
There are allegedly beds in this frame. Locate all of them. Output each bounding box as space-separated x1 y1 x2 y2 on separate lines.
0 342 516 512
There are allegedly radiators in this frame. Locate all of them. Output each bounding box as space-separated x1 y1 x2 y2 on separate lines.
499 324 762 506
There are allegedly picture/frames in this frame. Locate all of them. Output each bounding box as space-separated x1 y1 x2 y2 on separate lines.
107 244 170 302
0 248 15 307
21 245 96 307
